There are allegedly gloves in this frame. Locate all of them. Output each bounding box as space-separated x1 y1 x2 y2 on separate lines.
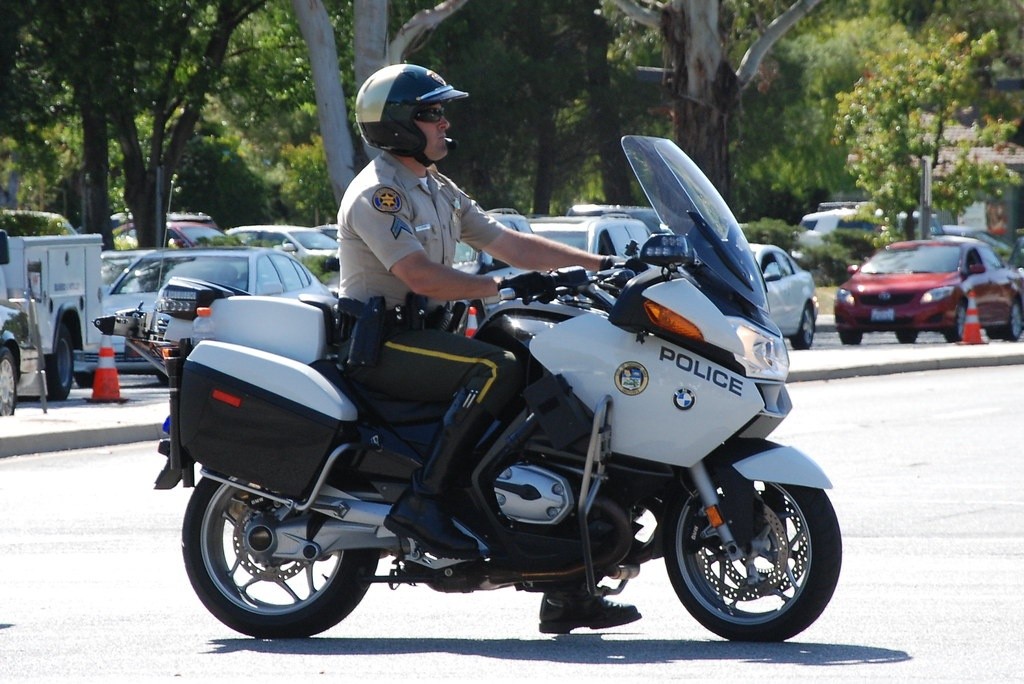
596 254 627 272
496 270 558 305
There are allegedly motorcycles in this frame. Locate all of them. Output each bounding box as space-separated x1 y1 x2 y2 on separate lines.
150 139 844 640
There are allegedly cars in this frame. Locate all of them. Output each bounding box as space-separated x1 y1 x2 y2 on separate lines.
832 232 1024 348
791 181 1016 263
0 191 821 422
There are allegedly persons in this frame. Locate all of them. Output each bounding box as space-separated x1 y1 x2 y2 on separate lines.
336 62 643 633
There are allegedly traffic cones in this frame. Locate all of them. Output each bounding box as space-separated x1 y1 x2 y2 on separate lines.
960 288 986 345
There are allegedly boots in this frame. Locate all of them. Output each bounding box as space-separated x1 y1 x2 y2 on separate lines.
383 388 483 562
538 593 642 634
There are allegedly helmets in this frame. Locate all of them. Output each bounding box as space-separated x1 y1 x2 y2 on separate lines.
352 64 469 156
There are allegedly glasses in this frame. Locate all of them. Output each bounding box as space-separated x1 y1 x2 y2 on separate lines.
415 108 445 123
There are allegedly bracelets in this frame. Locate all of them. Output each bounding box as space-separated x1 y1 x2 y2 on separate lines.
599 254 616 272
